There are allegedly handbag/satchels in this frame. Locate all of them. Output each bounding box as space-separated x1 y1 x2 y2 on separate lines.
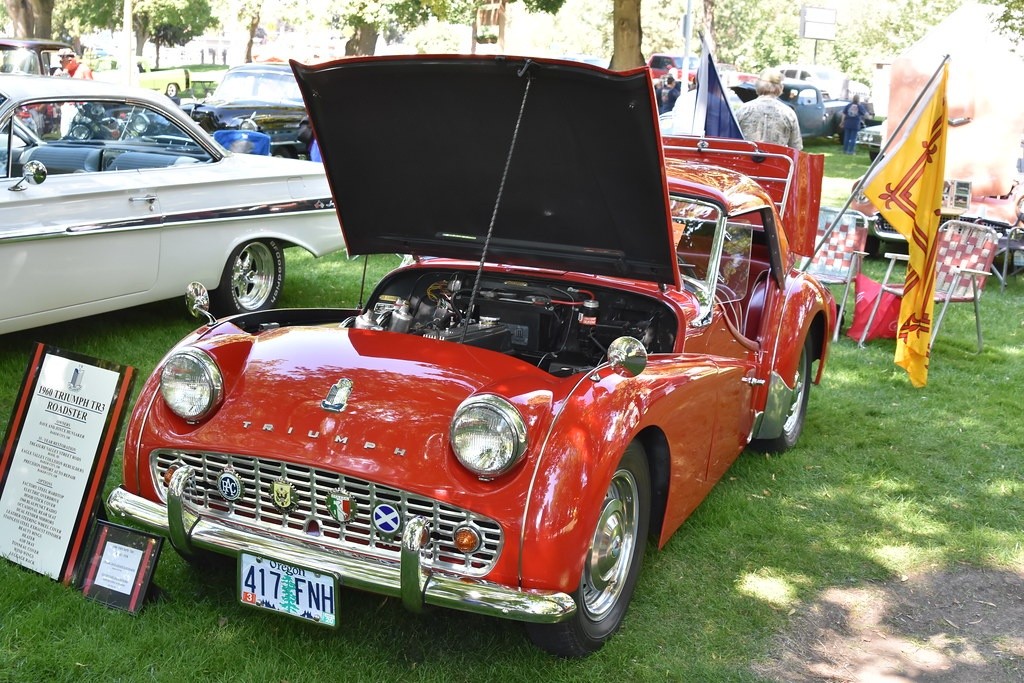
845 269 903 344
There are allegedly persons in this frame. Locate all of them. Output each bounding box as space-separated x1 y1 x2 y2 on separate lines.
55 48 96 136
735 68 804 152
839 95 870 156
653 66 697 116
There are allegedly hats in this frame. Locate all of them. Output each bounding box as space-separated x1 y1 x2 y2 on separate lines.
57 48 76 62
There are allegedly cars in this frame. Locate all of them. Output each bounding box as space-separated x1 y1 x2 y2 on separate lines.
854 124 884 162
122 54 835 654
646 54 852 145
0 71 346 336
0 37 314 162
850 173 1024 275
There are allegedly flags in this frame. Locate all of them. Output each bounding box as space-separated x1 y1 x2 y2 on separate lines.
863 64 950 387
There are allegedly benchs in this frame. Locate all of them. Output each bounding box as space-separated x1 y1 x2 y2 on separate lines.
678 234 773 342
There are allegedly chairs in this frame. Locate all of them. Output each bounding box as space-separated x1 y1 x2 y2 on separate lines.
12 144 214 178
857 219 998 355
973 192 1024 292
798 206 870 344
